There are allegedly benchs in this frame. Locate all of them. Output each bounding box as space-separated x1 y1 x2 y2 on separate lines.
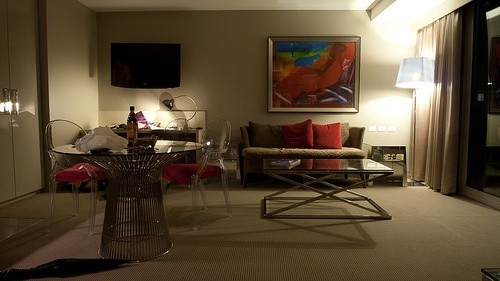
240 125 366 187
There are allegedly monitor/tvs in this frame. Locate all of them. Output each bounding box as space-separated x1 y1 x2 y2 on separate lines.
111 43 182 90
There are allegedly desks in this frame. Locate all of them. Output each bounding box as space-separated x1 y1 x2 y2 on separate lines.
80 111 206 164
52 139 204 262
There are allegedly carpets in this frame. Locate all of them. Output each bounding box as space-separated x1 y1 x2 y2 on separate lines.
0 187 500 281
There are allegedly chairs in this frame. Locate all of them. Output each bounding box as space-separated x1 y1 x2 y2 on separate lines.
161 118 192 141
160 120 232 233
45 119 108 235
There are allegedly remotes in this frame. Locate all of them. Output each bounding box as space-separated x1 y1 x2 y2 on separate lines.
89 148 111 153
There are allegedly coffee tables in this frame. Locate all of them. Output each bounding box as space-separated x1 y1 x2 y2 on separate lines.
263 159 394 219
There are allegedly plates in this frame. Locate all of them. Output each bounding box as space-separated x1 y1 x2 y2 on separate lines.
90 148 111 154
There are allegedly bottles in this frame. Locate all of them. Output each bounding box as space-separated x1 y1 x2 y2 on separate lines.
127 106 138 147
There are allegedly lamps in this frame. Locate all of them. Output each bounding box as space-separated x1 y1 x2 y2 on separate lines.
162 95 197 121
395 57 434 187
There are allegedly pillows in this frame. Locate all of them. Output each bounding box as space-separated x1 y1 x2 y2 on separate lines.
282 119 314 148
249 120 282 148
312 122 342 149
341 123 351 147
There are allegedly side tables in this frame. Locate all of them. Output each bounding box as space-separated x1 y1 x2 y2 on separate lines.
205 141 240 181
361 143 407 187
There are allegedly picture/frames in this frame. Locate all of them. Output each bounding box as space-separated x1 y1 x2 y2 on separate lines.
488 86 500 114
267 36 361 113
